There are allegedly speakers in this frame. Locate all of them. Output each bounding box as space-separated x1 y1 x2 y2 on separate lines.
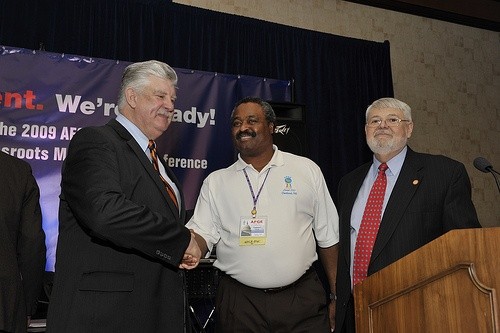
266 100 307 158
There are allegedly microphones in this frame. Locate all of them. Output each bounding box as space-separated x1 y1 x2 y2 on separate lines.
473 157 500 193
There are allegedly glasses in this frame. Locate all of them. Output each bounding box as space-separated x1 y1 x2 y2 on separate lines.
366 117 410 128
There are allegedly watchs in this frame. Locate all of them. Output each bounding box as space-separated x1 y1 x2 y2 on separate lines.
330 294 337 300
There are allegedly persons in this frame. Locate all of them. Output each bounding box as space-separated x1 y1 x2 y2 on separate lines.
0 150 47 333
178 96 340 333
328 97 481 333
49 61 201 333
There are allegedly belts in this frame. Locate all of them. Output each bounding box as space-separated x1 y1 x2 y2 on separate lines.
263 267 312 294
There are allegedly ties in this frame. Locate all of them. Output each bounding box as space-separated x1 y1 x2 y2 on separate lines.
146 139 179 212
352 163 388 286
